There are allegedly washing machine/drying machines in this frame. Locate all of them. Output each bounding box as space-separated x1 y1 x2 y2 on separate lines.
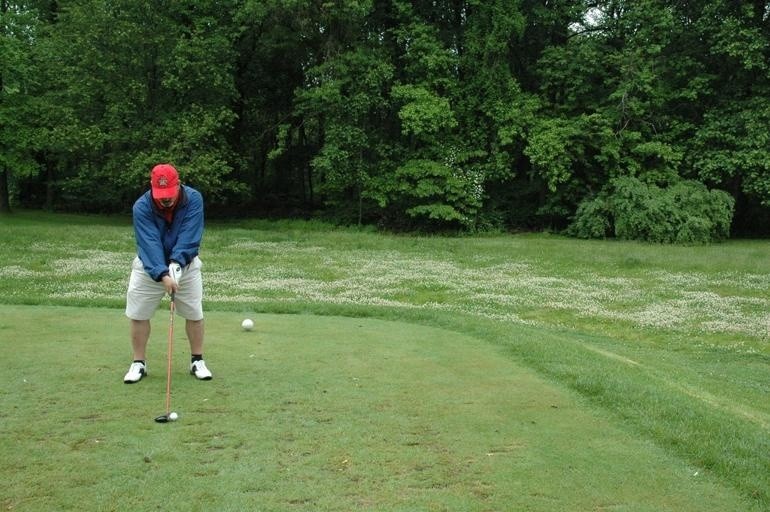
155 293 175 423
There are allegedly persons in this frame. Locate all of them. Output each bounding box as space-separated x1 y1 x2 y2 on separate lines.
125 165 212 383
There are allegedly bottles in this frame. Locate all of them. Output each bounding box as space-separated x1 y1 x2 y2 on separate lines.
151 164 179 199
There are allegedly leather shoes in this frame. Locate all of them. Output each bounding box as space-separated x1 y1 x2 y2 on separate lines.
169 263 183 285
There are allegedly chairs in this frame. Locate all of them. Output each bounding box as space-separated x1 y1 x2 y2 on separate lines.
124 362 147 383
189 360 212 380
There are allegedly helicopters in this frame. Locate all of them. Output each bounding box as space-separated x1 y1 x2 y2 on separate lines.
170 412 178 420
242 319 255 330
